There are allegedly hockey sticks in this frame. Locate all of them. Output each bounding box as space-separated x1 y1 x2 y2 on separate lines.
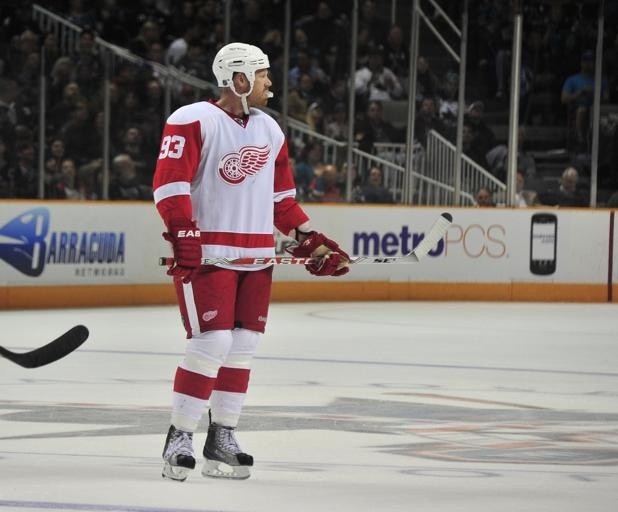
159 213 452 267
1 325 89 368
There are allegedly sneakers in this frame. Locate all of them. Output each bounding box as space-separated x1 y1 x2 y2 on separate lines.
203 423 254 466
162 424 196 469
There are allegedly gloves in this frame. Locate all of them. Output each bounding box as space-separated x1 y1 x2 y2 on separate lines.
162 219 202 283
284 229 350 277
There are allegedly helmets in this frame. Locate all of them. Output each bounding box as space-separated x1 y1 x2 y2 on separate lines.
212 43 271 95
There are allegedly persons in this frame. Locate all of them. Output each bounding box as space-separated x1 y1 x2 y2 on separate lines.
155 41 353 469
1 0 618 207
